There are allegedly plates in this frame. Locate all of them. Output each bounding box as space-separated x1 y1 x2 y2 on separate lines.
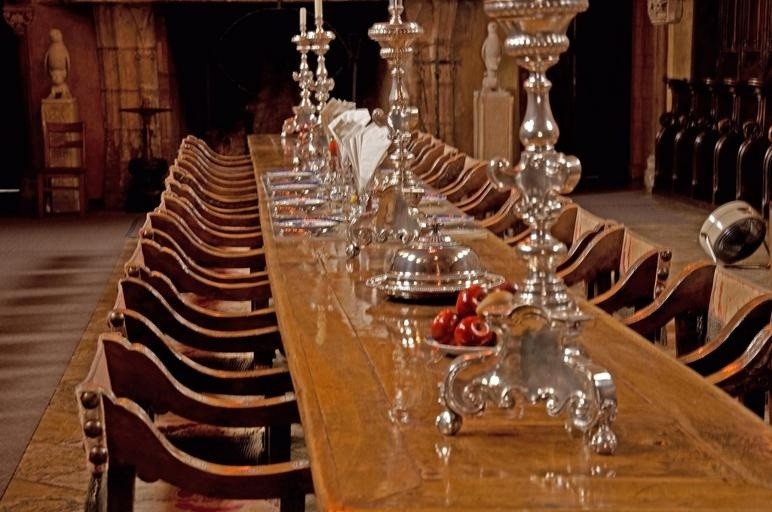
263 169 340 236
367 274 505 301
418 192 475 226
423 335 495 359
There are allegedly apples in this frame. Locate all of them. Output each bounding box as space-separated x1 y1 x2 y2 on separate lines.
431 281 519 346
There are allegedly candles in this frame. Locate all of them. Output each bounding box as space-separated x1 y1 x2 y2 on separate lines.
299 0 322 25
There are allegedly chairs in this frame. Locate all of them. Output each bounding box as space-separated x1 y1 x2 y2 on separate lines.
76 129 772 512
36 120 86 218
